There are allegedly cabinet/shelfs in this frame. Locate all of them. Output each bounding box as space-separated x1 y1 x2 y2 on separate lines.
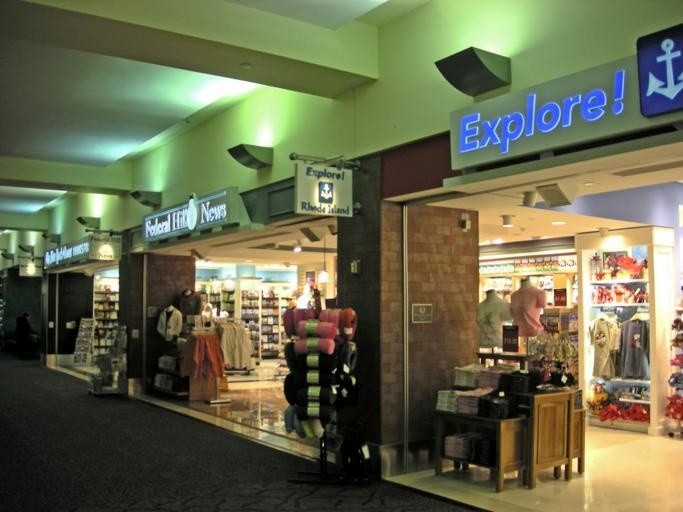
207 278 296 365
480 246 580 313
579 231 655 434
93 289 119 349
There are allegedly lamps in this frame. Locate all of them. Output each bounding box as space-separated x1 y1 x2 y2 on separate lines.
317 235 330 285
433 46 513 100
226 142 273 170
293 240 303 253
1 215 101 260
130 190 162 208
501 190 537 227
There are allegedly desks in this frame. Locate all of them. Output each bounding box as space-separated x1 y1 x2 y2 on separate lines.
479 351 533 371
434 386 587 492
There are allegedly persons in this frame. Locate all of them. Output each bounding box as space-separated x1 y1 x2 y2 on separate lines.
475 289 512 348
156 304 183 354
511 276 547 337
178 288 203 321
15 311 32 360
